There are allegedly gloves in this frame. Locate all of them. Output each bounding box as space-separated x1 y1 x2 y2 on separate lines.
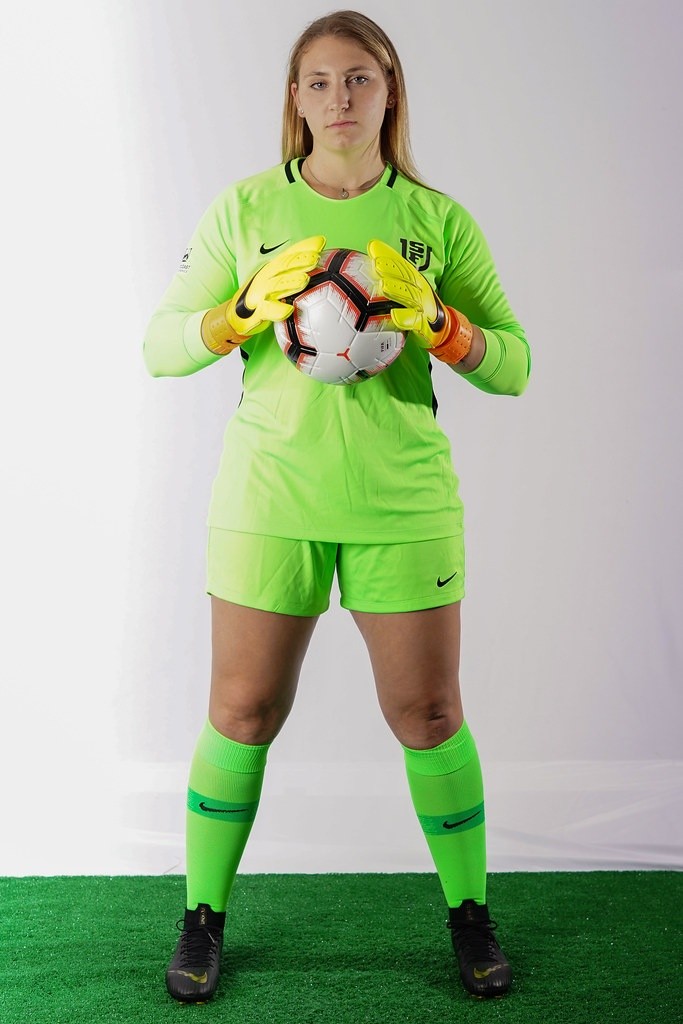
203 235 328 354
366 242 474 366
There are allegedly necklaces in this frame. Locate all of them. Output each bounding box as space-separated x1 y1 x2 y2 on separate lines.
306 157 387 200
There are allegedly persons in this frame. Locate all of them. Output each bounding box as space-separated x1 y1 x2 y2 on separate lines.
144 11 531 1003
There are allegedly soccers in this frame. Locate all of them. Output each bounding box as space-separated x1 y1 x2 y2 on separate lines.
268 247 411 386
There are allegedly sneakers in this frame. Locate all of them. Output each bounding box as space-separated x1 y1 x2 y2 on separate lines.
165 903 226 1006
445 899 514 999
